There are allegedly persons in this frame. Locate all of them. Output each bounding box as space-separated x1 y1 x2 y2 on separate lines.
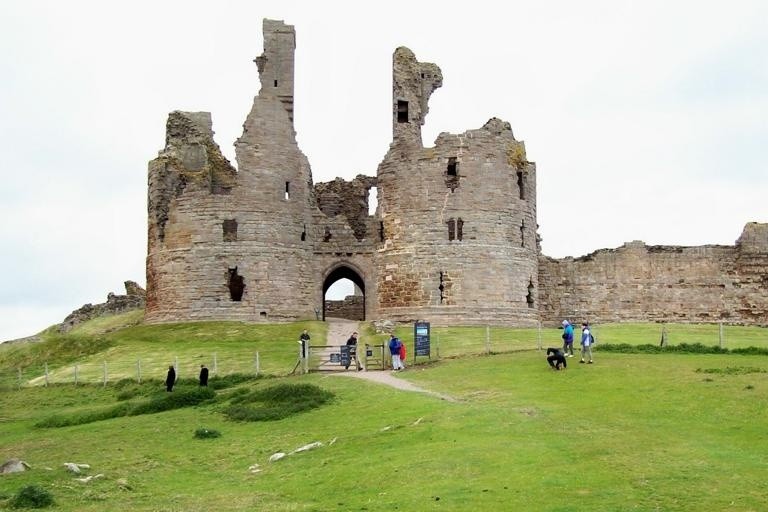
199 364 209 387
345 332 363 371
579 323 592 364
561 320 574 358
547 347 566 370
166 364 176 391
568 316 575 330
300 329 311 358
399 340 408 368
389 335 405 370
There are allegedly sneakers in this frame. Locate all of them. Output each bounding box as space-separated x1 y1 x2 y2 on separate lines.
563 352 594 364
